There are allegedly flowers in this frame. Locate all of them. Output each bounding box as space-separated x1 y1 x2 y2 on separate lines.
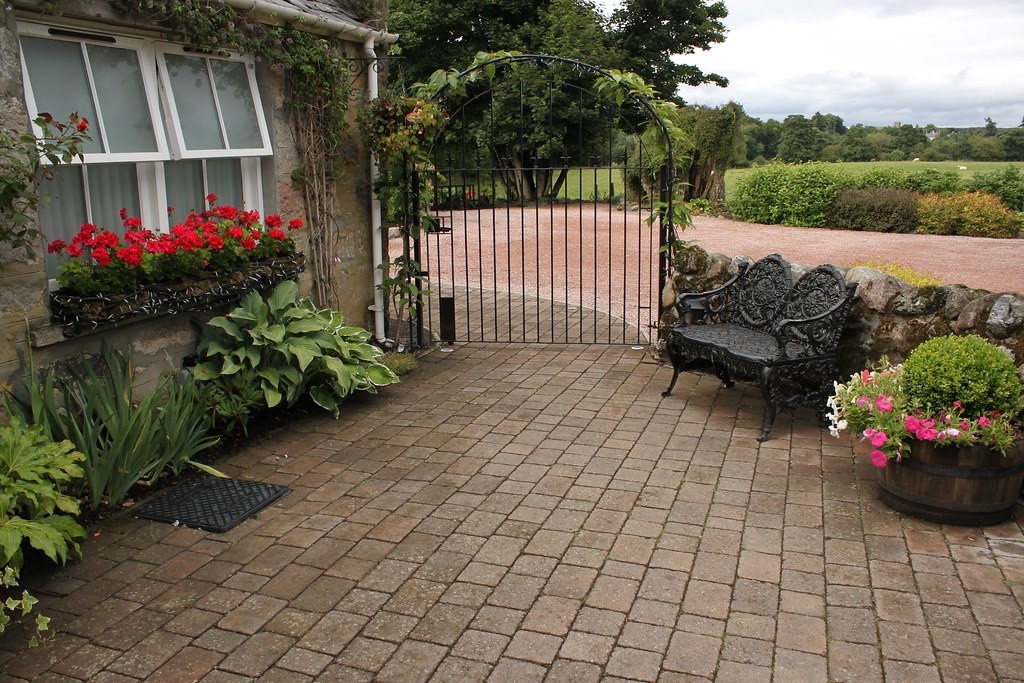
823 332 1024 469
47 192 303 292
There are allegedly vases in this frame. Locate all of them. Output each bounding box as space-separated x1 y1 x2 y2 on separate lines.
878 435 1024 527
49 252 307 340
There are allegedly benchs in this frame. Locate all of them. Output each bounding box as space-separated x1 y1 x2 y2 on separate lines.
661 253 859 441
